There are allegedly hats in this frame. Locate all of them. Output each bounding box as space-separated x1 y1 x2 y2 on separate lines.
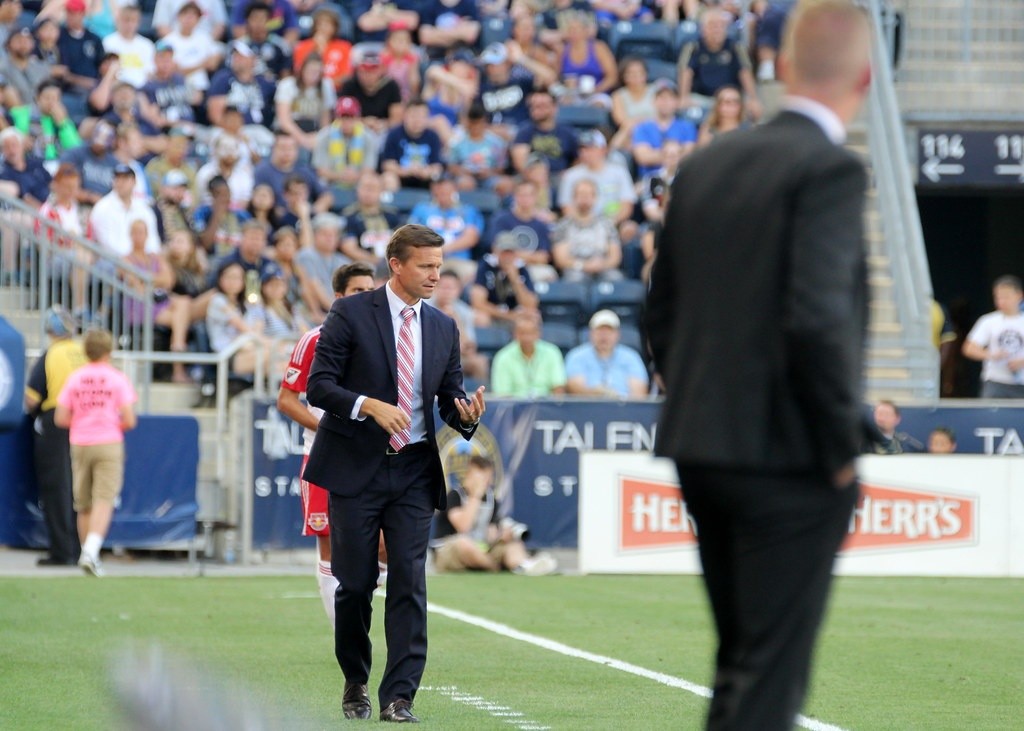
42 306 75 336
161 169 189 186
92 121 116 148
490 229 519 251
651 77 679 97
336 95 362 117
587 308 621 330
576 128 608 147
359 50 381 70
113 161 136 176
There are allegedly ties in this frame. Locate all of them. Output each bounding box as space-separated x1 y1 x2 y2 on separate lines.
389 306 416 452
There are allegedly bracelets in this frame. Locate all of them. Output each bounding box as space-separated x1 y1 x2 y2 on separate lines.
461 417 480 428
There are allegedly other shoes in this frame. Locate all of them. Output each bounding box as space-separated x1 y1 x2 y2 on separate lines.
38 556 78 567
78 552 103 578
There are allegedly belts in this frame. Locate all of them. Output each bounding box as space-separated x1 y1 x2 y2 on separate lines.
386 443 426 455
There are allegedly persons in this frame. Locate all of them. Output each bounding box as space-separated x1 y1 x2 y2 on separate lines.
928 427 957 454
636 0 874 731
276 264 387 629
490 310 568 397
563 310 649 400
24 304 91 565
0 0 782 378
961 275 1024 399
427 456 557 576
873 400 924 454
53 329 136 578
301 224 487 722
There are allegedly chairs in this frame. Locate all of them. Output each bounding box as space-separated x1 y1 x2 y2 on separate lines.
0 0 793 411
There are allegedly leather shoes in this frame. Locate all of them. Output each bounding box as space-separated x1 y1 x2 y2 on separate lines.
342 680 371 720
380 699 419 722
